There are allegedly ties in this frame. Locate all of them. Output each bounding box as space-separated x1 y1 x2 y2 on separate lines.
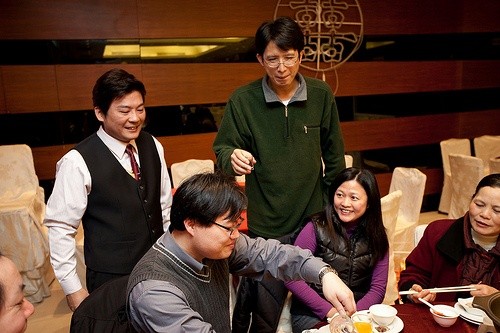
124 144 139 181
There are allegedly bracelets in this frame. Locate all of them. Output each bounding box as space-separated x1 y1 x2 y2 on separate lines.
318 267 338 282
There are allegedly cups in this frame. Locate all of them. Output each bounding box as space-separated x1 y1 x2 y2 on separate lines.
244 155 253 172
352 314 373 333
329 314 352 333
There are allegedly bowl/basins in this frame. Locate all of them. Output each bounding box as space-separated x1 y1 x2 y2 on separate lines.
430 305 459 327
369 303 397 326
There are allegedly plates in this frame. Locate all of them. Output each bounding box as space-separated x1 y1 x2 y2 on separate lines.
350 309 404 333
454 296 489 327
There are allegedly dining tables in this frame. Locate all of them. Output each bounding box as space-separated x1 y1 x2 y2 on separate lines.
172 181 250 232
334 302 500 333
488 155 499 174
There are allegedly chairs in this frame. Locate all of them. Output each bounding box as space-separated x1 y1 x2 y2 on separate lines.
171 158 215 187
381 191 403 303
439 133 500 219
389 168 427 260
0 145 55 300
322 155 352 175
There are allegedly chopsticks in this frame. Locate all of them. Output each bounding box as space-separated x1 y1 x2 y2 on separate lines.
398 285 477 294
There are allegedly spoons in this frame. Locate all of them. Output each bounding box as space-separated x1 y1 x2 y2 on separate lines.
418 297 457 318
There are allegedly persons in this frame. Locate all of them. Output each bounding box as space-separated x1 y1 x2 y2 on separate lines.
0 253 36 333
211 15 349 333
397 173 500 304
282 166 390 333
125 168 356 333
43 68 174 333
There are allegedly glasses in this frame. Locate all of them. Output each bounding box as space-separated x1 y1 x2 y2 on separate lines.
211 217 244 235
262 56 299 66
